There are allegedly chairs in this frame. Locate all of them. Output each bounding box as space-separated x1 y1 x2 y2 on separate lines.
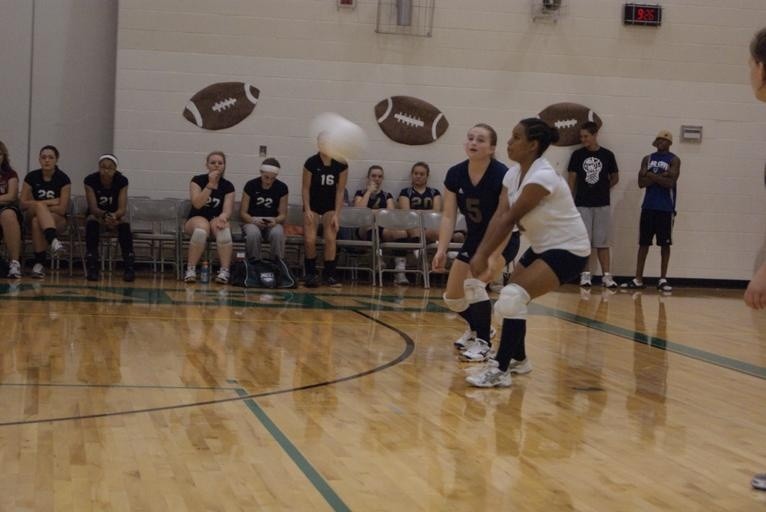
22 194 469 289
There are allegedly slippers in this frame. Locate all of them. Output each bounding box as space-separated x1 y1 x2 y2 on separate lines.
622 280 645 289
656 282 674 292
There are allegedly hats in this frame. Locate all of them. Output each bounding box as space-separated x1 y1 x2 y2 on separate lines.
653 130 672 145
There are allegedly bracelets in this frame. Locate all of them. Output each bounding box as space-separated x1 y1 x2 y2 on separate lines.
205 186 213 191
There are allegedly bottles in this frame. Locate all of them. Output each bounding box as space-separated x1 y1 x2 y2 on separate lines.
200 261 210 283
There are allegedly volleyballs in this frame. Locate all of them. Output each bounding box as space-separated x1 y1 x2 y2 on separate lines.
310 112 367 162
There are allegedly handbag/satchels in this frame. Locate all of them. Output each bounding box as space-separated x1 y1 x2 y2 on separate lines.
232 256 298 289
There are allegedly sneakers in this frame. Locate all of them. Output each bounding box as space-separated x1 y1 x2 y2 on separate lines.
323 277 343 288
215 269 232 283
123 271 136 281
7 260 21 278
304 275 321 288
394 271 409 285
459 338 495 361
466 358 511 388
580 272 592 286
490 356 531 375
85 269 99 280
51 240 68 260
30 264 45 278
601 271 618 289
455 326 496 349
185 266 197 282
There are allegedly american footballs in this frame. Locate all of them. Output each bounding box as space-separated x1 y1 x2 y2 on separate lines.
535 103 603 146
183 81 260 130
376 95 449 145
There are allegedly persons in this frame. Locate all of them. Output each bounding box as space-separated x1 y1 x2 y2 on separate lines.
17 144 71 280
743 26 766 312
0 142 23 280
300 130 350 289
564 121 621 290
620 129 683 293
182 150 236 285
430 122 522 364
83 153 137 282
460 116 594 390
238 157 290 265
351 164 412 286
396 161 443 260
